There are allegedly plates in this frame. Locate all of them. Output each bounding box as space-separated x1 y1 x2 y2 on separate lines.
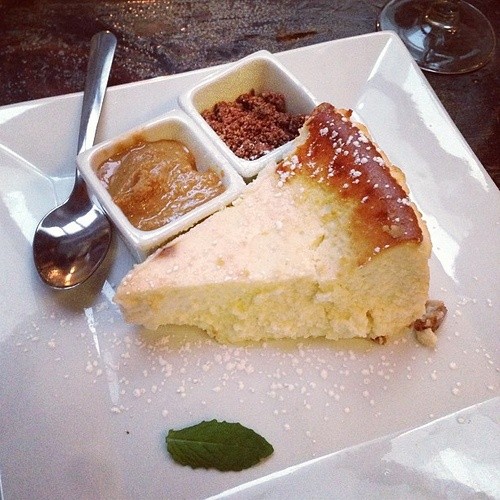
0 30 500 500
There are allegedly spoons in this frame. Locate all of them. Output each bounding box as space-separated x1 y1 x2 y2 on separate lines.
33 32 117 291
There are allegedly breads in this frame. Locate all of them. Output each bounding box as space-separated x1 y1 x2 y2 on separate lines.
111 102 434 342
